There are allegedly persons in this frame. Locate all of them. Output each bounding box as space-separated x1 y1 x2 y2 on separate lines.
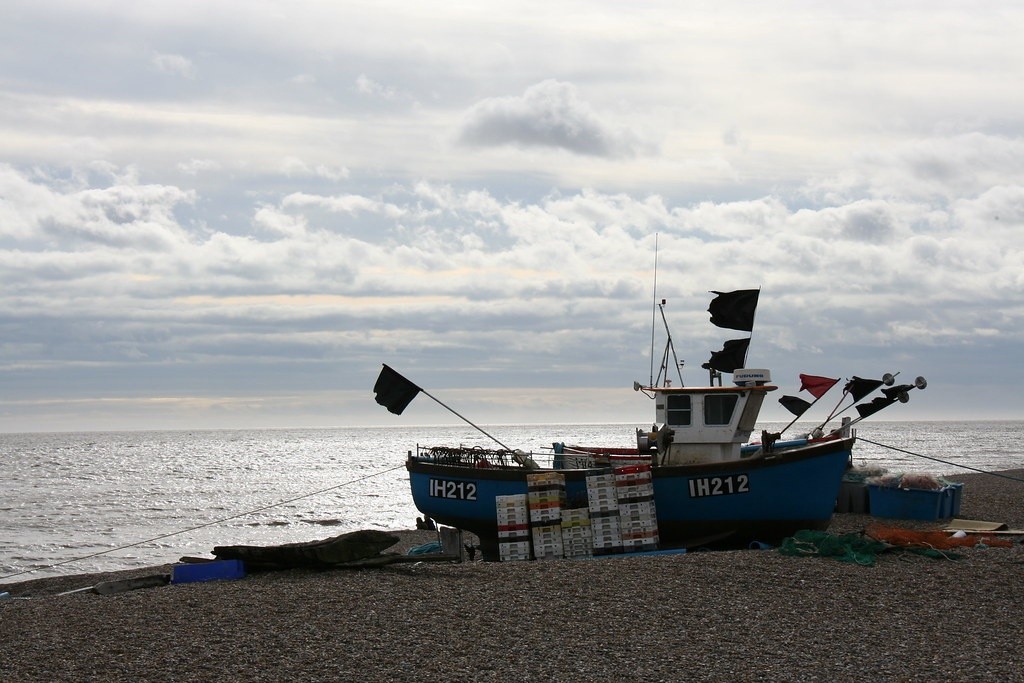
424 515 435 530
416 517 428 530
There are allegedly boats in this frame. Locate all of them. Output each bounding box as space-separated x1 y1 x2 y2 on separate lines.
371 235 928 547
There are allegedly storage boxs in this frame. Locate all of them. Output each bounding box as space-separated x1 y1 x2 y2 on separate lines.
495 464 661 562
834 481 964 522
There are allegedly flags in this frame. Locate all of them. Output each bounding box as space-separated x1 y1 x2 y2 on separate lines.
372 366 422 416
778 395 810 416
799 374 837 398
702 338 749 373
707 289 758 332
842 376 914 417
